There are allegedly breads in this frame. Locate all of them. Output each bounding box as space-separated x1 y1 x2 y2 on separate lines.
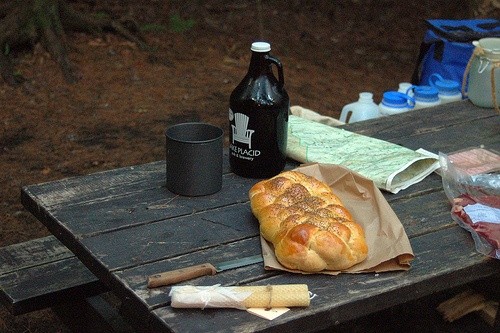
249 170 368 272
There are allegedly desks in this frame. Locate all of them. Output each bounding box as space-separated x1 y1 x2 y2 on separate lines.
20 98 500 333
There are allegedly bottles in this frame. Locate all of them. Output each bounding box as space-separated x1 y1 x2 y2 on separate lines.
339 92 379 123
228 42 290 180
378 91 416 117
397 82 413 98
468 37 500 108
428 73 463 104
406 86 442 111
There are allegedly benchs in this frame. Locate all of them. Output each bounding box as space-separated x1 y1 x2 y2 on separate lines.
1 236 137 333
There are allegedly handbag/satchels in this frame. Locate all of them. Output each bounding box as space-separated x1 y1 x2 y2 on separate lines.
411 18 500 98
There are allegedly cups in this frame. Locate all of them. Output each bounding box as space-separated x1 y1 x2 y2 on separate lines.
165 122 224 197
